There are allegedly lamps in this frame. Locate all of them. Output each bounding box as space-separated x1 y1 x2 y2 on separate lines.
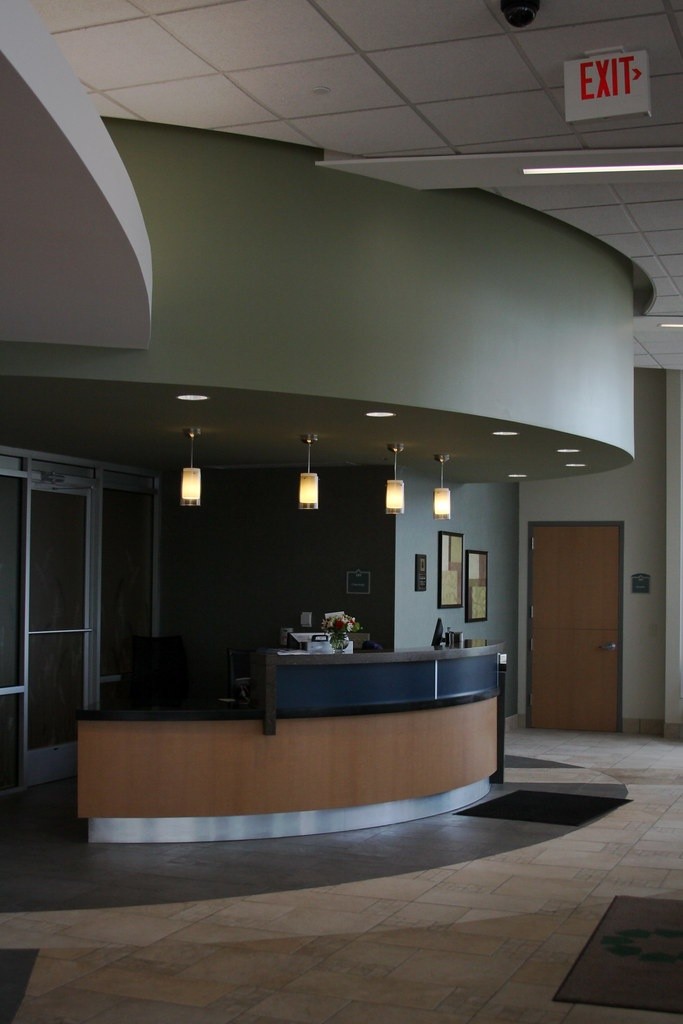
299 435 319 511
180 426 201 506
385 445 406 514
432 454 453 521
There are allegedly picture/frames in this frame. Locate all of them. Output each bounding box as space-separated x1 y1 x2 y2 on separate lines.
465 549 489 623
438 531 464 609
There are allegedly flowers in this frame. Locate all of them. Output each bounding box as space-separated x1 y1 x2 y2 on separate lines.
321 614 360 634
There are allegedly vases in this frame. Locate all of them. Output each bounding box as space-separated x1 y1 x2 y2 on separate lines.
329 633 349 653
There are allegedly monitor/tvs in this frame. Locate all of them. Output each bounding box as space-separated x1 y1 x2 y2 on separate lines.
431 618 450 648
287 633 332 651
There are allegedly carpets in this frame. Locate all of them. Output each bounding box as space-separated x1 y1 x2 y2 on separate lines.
552 895 683 1014
454 789 634 826
0 948 40 1024
